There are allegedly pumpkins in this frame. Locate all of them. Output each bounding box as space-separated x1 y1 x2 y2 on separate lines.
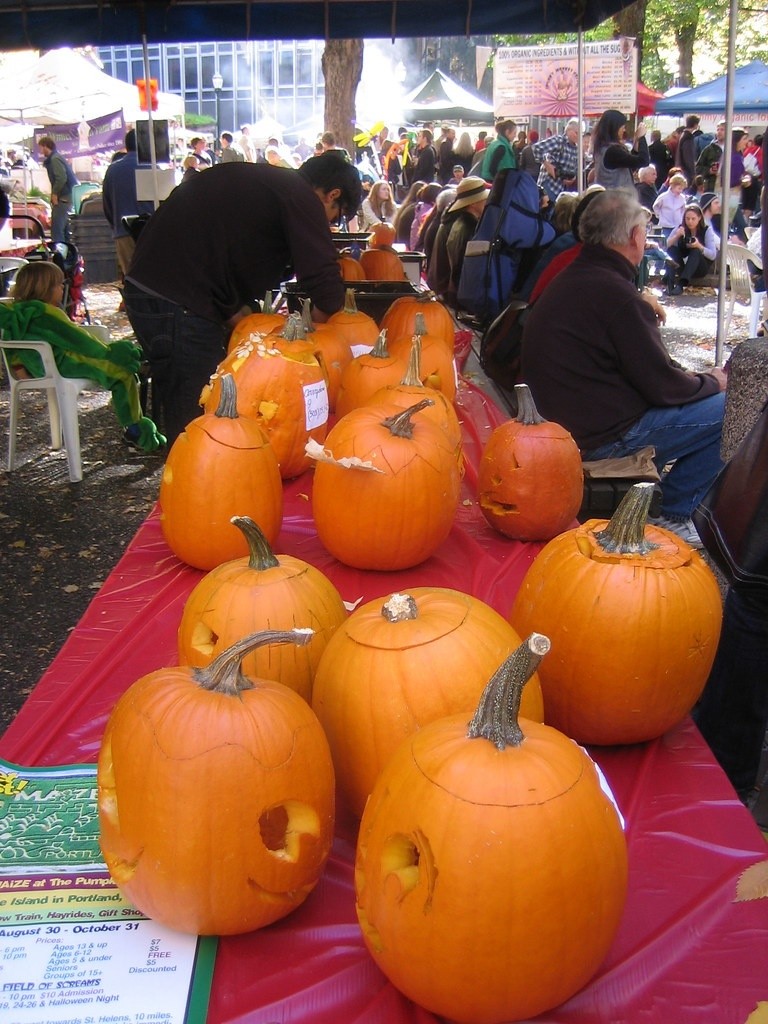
338 219 405 282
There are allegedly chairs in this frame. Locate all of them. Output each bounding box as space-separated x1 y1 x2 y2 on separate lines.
578 448 665 525
0 337 101 483
72 182 101 215
634 235 676 297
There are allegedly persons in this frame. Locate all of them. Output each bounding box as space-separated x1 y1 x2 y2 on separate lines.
170 123 359 235
101 131 162 272
689 395 768 804
123 155 363 446
361 110 768 548
0 149 28 230
38 137 82 242
0 261 167 454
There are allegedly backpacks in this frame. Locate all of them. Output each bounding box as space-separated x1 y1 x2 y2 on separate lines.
480 300 530 378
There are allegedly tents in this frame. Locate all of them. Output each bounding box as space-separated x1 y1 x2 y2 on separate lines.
542 60 768 135
403 70 495 122
0 47 184 194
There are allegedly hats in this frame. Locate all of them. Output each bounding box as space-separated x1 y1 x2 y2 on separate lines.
435 188 458 212
452 165 464 171
700 193 717 211
448 175 491 212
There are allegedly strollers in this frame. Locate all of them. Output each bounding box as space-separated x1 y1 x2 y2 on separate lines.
0 214 91 326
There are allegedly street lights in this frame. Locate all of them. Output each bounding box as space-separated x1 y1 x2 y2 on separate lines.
212 71 223 149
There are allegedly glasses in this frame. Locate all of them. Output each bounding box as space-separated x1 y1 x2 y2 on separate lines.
330 195 342 227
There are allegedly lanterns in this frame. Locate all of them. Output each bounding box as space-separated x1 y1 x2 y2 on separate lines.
355 711 627 1024
94 330 350 938
478 418 583 541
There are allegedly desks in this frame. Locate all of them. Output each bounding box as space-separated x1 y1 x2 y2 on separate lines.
0 330 768 1024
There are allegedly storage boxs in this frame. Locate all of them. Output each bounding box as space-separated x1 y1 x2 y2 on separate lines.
280 280 420 329
397 250 427 286
330 231 375 252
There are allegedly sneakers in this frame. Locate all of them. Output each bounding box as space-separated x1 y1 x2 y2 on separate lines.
121 427 143 450
647 512 705 548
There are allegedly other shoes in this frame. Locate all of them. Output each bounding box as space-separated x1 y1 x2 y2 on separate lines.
666 279 674 295
671 285 683 294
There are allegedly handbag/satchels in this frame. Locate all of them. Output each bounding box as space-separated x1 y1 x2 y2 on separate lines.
743 147 761 177
690 400 767 594
